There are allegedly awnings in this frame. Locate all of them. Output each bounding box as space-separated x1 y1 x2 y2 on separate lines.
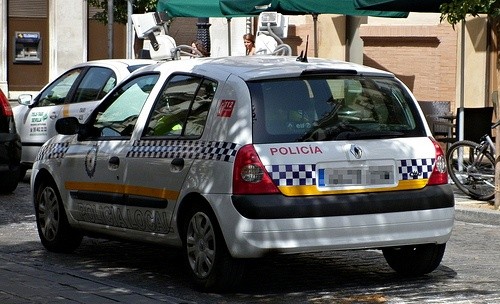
156 0 410 58
353 0 500 172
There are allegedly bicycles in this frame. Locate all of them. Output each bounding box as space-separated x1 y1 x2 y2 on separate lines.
447 120 499 204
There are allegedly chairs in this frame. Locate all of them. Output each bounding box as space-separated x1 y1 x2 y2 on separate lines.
433 107 496 163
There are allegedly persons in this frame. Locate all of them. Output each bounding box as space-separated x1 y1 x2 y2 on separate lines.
243 33 255 56
191 39 207 58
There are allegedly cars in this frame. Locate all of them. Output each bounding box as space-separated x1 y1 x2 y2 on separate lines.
27 34 458 284
1 88 22 196
12 57 162 185
